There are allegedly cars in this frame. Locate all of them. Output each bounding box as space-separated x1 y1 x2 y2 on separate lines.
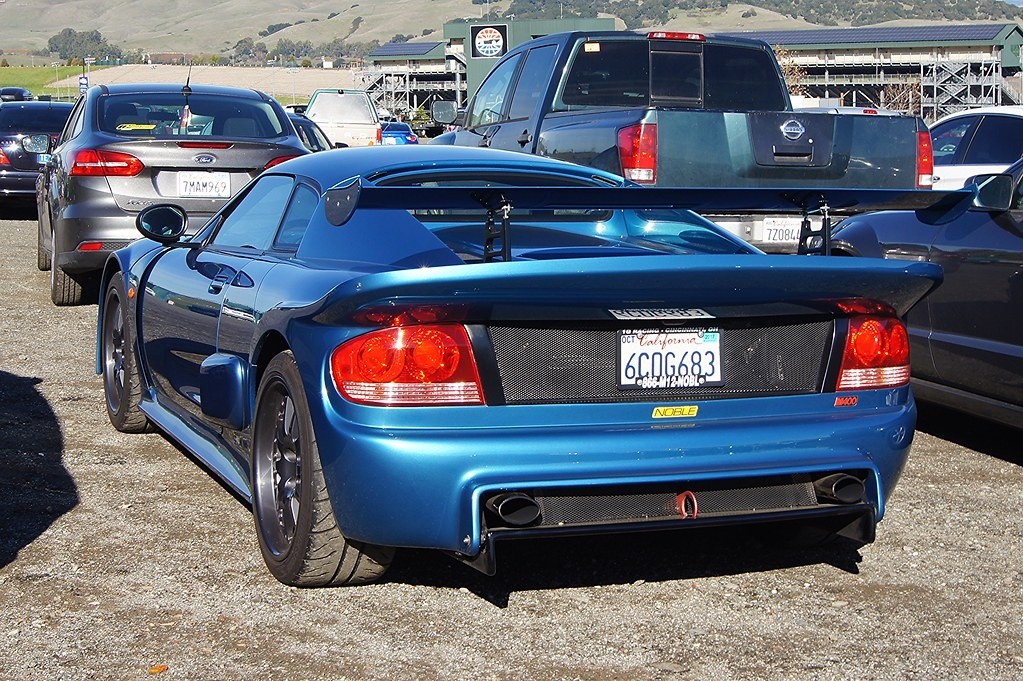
22 84 348 306
0 87 77 209
381 122 418 146
799 106 1023 430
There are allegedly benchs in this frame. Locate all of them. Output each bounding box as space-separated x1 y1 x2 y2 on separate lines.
116 116 259 139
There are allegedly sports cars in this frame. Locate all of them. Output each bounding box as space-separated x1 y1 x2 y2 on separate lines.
97 145 917 589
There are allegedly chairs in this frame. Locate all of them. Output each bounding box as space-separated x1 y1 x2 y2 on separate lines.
104 104 137 131
213 113 240 134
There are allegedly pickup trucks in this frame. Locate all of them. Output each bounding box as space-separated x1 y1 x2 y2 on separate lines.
428 32 934 254
303 89 382 149
